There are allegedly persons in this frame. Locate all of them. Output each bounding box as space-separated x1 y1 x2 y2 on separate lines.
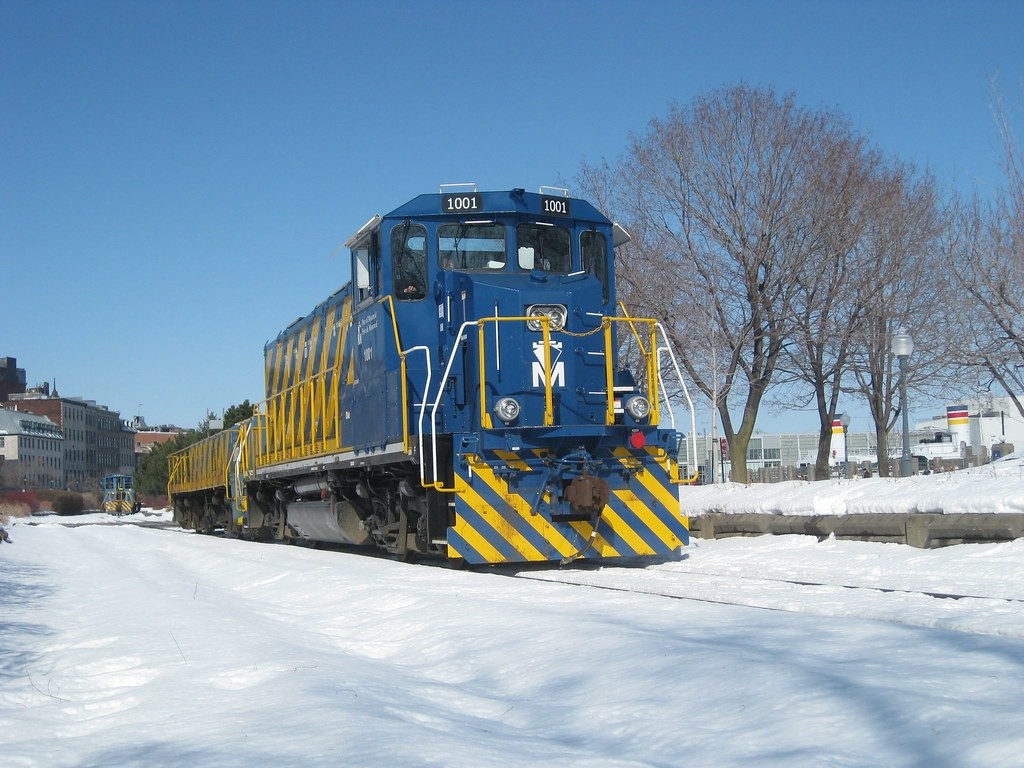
394 248 425 300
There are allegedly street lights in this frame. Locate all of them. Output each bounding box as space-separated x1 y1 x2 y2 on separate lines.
779 435 783 466
796 435 801 467
839 411 852 480
890 323 914 477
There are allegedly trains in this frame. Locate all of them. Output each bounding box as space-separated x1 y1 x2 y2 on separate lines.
102 472 141 516
165 182 699 566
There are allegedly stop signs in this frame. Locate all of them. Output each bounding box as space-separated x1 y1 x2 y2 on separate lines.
721 439 726 454
